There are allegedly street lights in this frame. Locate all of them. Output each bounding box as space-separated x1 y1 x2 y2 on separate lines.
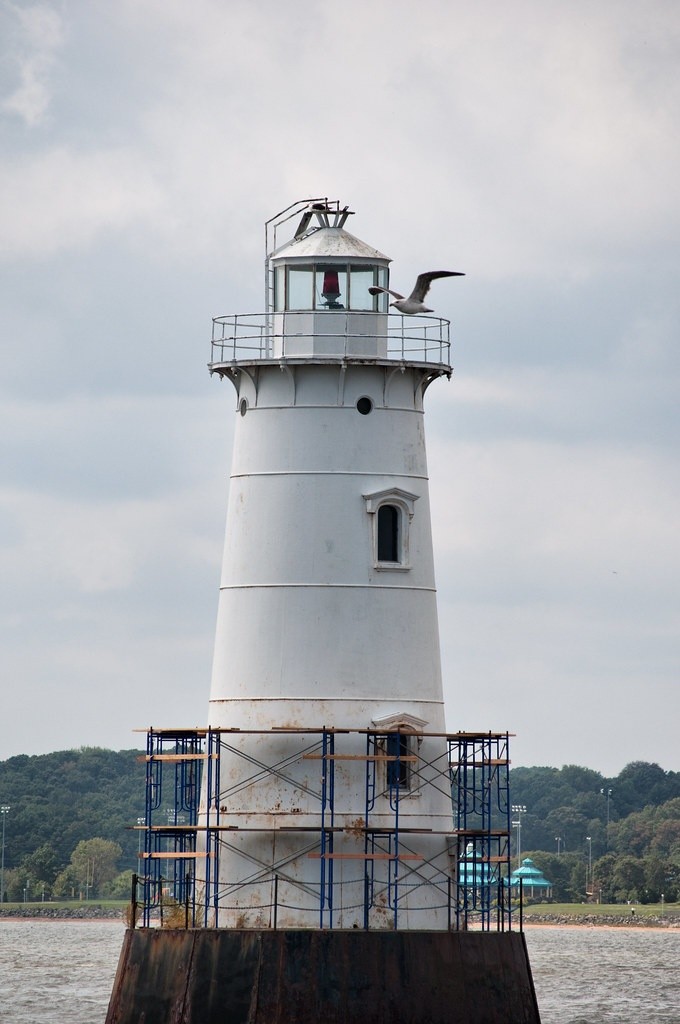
586 837 592 882
600 788 612 854
555 836 561 857
512 805 527 868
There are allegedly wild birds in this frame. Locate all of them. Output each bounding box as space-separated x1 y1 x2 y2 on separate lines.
368 270 466 315
